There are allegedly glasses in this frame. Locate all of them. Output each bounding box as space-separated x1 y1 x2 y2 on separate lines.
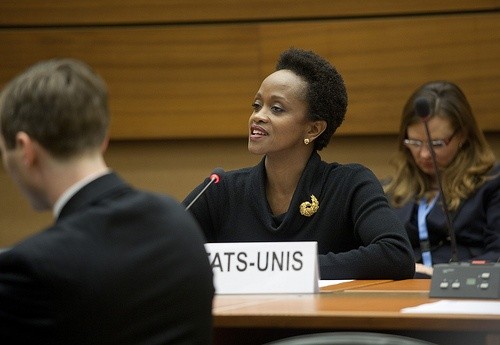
403 127 460 150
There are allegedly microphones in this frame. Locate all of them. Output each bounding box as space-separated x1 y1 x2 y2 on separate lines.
416 97 459 264
185 168 225 211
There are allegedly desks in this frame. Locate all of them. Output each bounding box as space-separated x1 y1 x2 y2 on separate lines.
211 279 500 345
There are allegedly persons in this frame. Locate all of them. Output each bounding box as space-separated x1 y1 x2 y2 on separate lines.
379 80 500 279
180 46 416 280
1 57 217 345
